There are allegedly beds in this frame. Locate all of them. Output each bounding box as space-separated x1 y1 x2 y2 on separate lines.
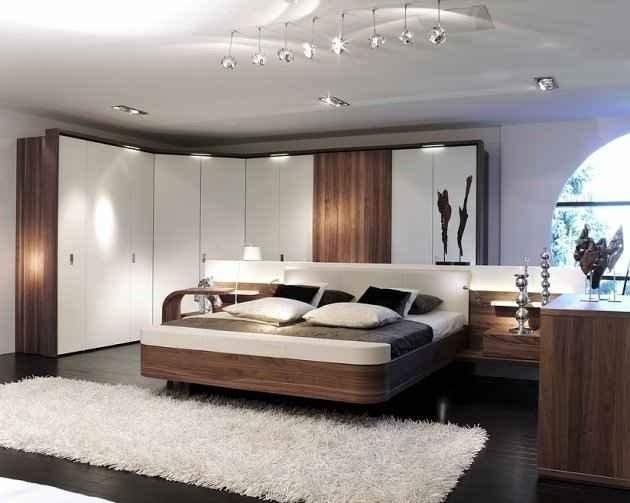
138 262 471 406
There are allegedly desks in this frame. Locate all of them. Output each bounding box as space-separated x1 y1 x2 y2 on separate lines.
161 287 260 325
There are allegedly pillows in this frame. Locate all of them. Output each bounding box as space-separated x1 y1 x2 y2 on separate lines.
222 283 445 329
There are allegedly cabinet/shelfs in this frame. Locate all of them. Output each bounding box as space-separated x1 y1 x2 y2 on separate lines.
313 145 392 263
153 149 246 324
536 292 630 490
246 150 314 284
14 128 154 359
392 140 488 265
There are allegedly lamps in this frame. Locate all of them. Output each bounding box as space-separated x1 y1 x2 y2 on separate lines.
216 0 452 71
234 246 265 305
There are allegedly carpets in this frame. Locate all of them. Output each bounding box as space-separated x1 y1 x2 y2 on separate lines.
0 375 491 503
